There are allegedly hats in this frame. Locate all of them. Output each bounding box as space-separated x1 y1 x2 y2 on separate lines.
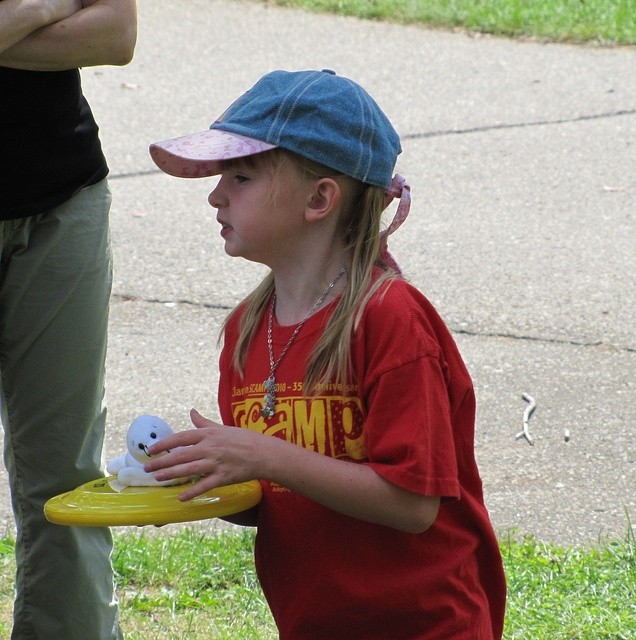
150 69 411 253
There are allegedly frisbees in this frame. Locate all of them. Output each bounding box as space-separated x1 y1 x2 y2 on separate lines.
43 474 263 526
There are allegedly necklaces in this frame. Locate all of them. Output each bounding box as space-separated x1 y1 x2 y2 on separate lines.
261 267 345 418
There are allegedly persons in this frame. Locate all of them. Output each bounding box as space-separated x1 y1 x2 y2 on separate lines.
0 0 138 640
142 69 506 636
